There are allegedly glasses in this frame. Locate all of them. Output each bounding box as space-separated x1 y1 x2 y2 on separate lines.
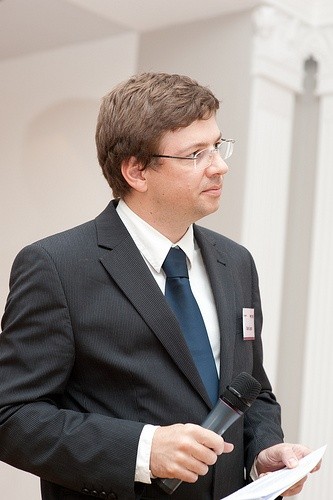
151 138 235 170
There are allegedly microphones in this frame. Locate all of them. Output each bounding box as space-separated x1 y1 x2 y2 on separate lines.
156 372 260 494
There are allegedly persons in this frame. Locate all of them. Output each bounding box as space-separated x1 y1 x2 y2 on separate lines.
0 73 323 500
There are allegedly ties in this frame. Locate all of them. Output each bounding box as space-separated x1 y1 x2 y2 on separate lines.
160 247 219 408
